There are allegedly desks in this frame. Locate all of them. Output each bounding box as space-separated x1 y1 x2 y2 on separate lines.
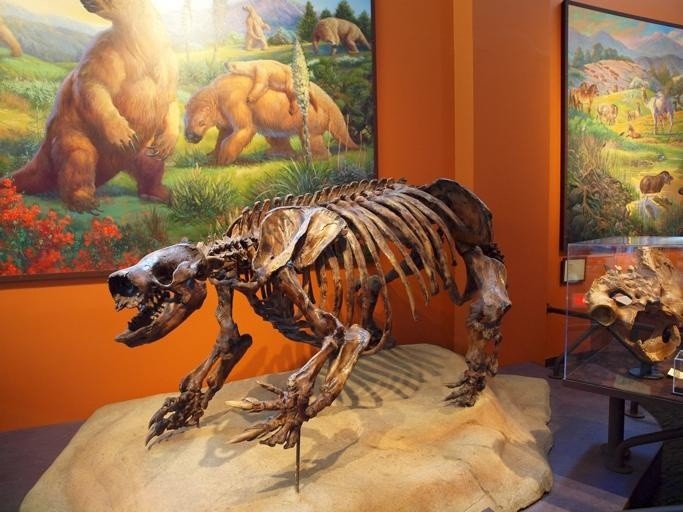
561 336 682 473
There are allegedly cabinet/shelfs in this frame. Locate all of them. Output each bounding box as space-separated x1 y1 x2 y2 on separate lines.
563 236 683 402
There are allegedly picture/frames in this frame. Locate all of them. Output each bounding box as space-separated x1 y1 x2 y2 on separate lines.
560 0 683 260
560 257 586 285
2 0 378 288
